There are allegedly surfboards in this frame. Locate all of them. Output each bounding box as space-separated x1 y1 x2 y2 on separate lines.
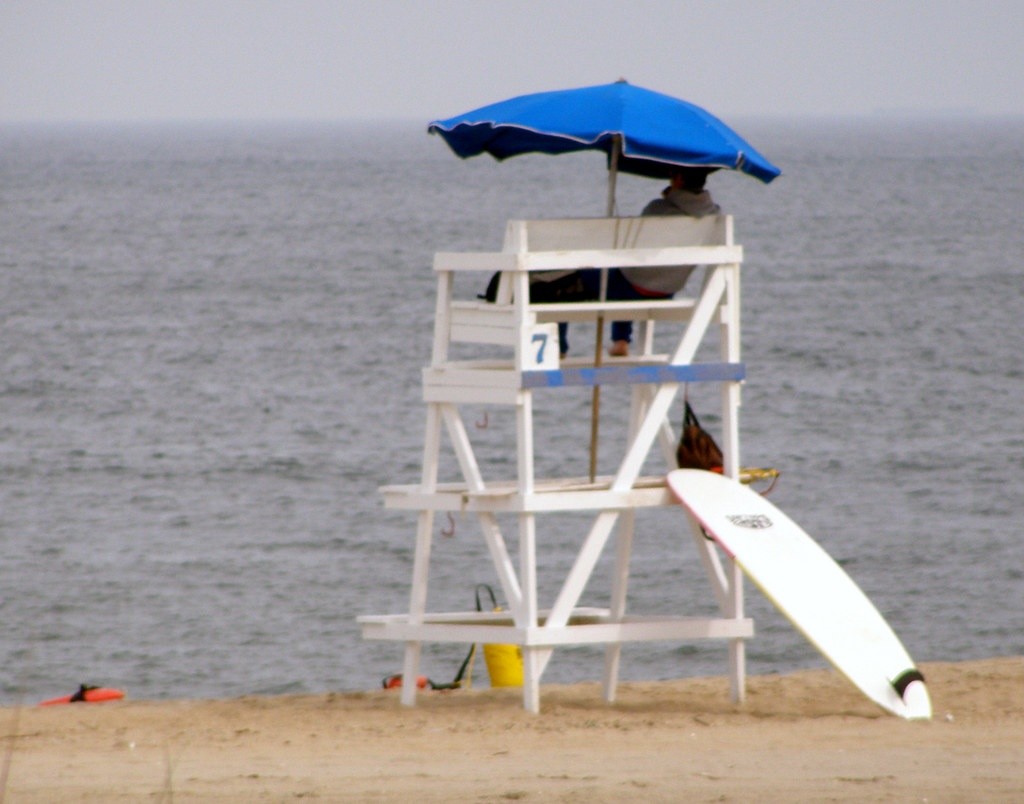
664 460 937 727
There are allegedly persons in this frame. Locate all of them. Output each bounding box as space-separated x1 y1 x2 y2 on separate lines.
530 166 720 359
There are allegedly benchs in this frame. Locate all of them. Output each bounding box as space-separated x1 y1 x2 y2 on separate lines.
449 211 722 346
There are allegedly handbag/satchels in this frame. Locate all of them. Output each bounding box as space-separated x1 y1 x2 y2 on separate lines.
676 401 723 471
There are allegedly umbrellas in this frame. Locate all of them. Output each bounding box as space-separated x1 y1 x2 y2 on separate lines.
428 78 784 484
